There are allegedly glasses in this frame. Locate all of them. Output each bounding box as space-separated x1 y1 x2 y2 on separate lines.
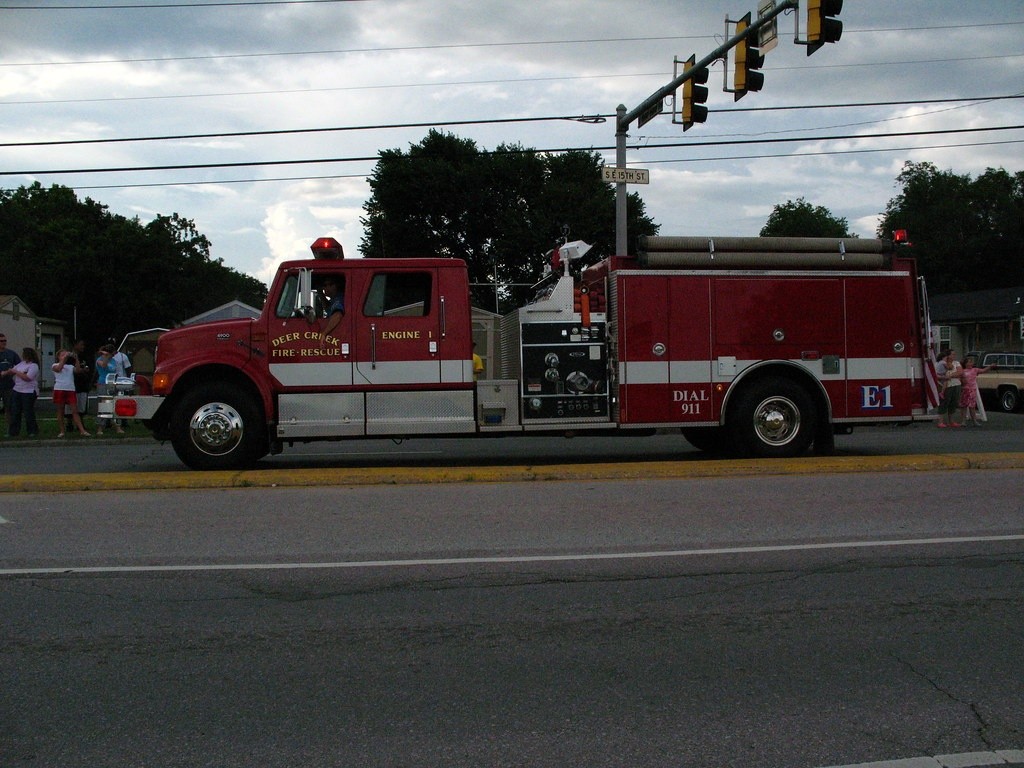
0 340 7 342
112 354 114 356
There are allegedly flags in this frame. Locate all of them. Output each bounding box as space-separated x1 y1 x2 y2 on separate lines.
919 279 940 410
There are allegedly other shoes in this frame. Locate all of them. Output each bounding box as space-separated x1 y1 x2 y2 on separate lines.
77 428 87 431
105 423 112 428
57 432 64 437
4 434 10 437
939 392 945 399
68 427 73 431
28 433 36 437
961 421 967 427
80 432 91 436
121 424 129 428
972 422 981 427
96 430 103 434
947 423 961 427
117 428 124 433
937 423 947 427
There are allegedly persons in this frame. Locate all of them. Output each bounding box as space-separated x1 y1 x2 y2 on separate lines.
0 333 132 439
935 348 997 428
316 276 345 349
473 340 484 381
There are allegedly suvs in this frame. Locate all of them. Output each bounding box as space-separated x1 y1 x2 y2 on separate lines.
960 349 1024 414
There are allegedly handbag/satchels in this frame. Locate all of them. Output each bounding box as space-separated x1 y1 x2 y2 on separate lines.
74 372 90 394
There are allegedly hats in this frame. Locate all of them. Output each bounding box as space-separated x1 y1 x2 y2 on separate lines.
108 336 118 343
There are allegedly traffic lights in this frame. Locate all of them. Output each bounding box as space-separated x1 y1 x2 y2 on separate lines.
807 0 844 57
681 53 709 132
734 10 766 92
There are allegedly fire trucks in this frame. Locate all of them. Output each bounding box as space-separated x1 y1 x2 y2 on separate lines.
96 238 943 460
